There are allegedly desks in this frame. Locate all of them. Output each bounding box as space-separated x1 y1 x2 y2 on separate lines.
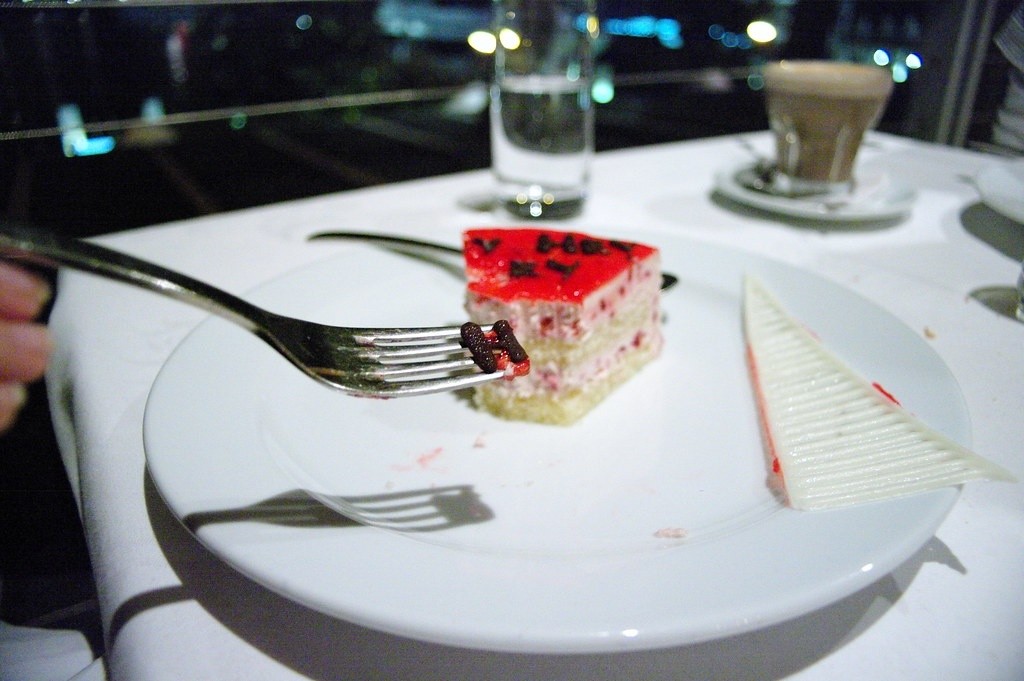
53 125 1024 681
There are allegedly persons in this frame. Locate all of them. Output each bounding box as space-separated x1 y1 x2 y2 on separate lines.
0 262 55 429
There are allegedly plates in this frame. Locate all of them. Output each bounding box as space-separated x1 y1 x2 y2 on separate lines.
143 225 971 654
976 166 1024 224
709 160 916 222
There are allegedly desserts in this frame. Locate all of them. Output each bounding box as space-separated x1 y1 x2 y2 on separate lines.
462 227 662 426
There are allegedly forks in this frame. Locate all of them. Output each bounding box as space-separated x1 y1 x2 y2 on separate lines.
1 227 506 396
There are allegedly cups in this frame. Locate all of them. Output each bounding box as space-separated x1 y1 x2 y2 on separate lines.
492 0 593 221
765 61 892 198
